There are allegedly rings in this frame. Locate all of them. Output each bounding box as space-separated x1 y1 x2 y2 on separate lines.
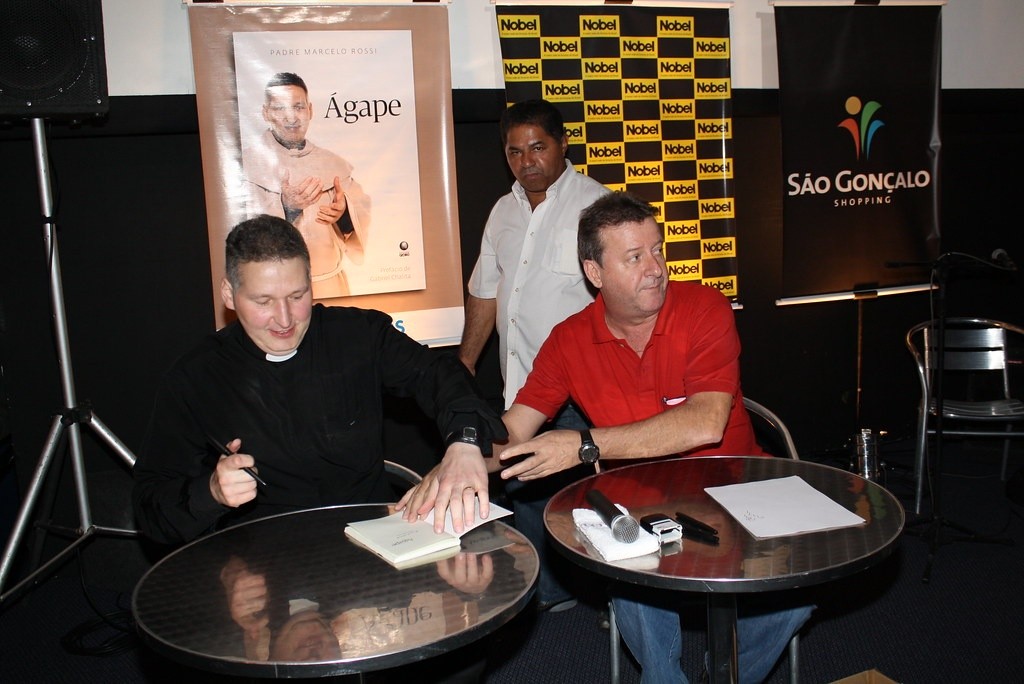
464 487 475 490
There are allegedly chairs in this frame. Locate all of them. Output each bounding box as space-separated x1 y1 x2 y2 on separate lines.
592 398 800 684
905 317 1024 515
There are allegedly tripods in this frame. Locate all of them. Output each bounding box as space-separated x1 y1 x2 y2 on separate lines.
0 116 136 602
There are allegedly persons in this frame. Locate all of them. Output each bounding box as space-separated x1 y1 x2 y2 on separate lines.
484 190 818 684
242 72 372 299
546 459 749 582
129 214 512 582
461 98 614 413
191 506 526 664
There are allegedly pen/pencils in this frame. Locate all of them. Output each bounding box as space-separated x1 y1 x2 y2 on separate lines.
205 432 267 487
675 511 719 535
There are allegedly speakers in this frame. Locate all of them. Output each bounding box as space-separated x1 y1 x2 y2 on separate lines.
1 0 108 119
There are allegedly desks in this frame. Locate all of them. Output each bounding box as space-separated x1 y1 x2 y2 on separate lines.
545 454 906 684
133 501 541 684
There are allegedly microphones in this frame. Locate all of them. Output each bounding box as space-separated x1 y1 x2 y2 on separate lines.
586 489 639 543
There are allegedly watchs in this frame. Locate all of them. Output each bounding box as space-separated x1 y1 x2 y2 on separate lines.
446 428 485 452
579 429 601 464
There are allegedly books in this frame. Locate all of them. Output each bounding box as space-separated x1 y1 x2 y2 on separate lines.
344 494 514 564
